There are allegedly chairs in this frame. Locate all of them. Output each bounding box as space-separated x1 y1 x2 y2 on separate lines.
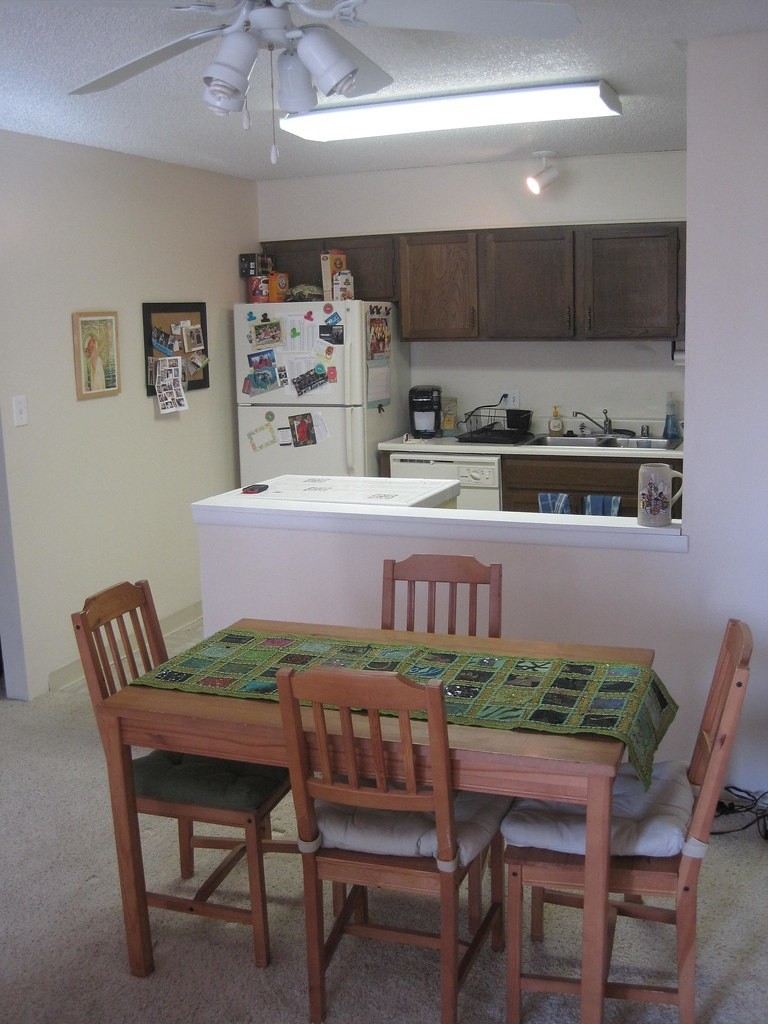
500 619 754 1024
382 553 502 639
276 664 515 1023
72 579 291 978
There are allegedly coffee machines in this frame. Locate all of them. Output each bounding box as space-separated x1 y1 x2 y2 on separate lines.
408 385 443 440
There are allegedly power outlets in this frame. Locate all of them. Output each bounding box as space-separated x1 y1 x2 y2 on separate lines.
501 390 520 409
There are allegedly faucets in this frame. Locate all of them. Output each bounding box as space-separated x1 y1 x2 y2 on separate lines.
573 409 612 436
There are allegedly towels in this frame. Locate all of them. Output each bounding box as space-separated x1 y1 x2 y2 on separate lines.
537 493 572 514
582 495 622 516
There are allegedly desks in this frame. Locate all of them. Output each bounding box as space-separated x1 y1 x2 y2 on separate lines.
100 618 678 1024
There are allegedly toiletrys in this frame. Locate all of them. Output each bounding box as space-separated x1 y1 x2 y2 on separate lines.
548 405 563 436
662 391 681 438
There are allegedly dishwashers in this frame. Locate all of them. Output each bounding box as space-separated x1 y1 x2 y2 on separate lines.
390 452 503 511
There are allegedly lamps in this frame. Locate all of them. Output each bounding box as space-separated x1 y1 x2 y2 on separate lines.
201 9 360 164
278 80 622 141
526 151 559 195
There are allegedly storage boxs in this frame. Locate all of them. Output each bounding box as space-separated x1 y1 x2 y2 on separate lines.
238 253 272 276
269 273 289 302
321 249 354 300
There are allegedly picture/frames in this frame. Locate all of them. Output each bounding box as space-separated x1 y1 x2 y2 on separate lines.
72 312 121 401
142 302 210 396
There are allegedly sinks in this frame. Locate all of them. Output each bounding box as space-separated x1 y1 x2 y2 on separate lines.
525 437 603 447
598 439 667 448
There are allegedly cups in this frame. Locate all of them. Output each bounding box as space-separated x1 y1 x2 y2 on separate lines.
637 463 683 527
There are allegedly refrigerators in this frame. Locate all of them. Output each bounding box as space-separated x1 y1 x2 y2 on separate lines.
232 301 410 488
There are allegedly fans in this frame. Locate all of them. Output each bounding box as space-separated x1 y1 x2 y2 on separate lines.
68 0 582 96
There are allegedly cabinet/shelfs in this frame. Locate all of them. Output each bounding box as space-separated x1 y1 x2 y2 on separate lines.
482 222 686 342
501 454 683 519
259 235 396 304
396 230 481 343
